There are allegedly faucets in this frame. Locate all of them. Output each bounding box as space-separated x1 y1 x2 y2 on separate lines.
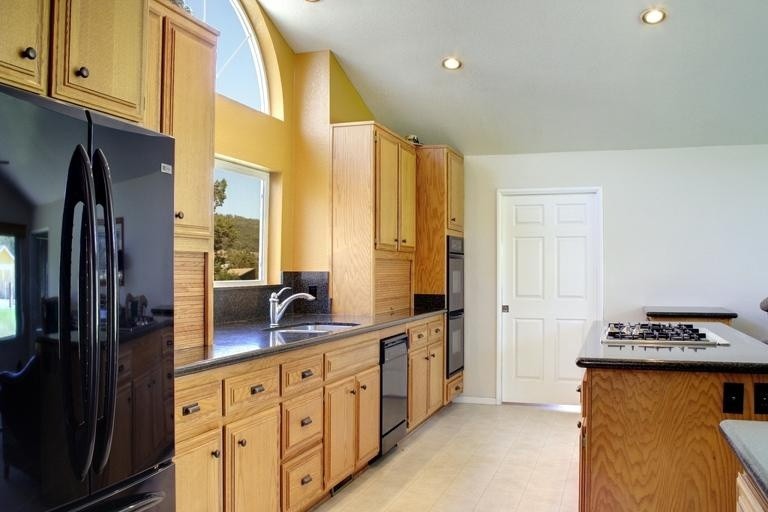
268 286 317 329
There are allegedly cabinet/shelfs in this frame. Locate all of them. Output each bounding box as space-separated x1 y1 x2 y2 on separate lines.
331 121 416 315
407 319 443 429
174 364 282 512
280 339 381 512
417 144 464 233
0 1 149 123
141 0 221 352
447 376 464 401
575 305 768 512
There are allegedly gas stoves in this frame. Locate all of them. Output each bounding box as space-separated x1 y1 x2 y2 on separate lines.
600 321 731 343
601 344 730 353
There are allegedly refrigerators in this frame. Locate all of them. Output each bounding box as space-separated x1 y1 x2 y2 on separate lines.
0 86 177 512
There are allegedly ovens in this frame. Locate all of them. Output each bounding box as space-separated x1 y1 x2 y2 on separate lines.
378 332 410 458
446 311 464 381
446 235 465 312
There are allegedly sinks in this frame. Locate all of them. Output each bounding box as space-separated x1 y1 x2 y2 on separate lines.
265 320 360 334
